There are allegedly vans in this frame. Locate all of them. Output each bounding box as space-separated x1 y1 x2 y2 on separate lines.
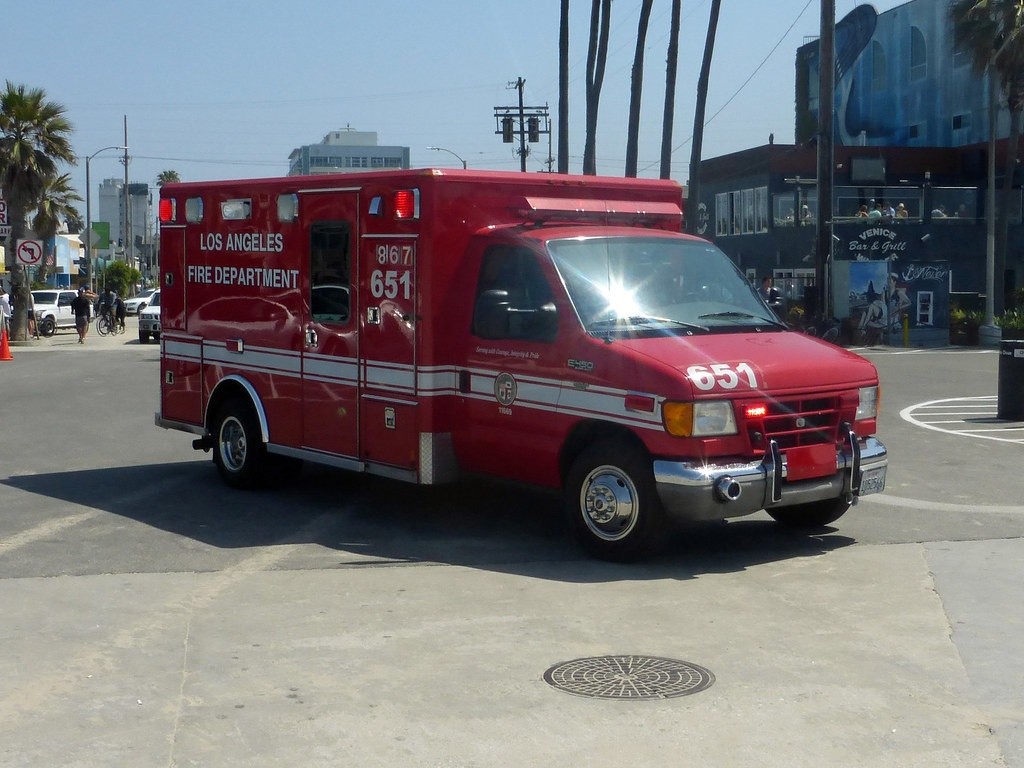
30 289 96 336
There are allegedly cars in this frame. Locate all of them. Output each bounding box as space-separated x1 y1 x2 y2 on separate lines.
120 286 162 317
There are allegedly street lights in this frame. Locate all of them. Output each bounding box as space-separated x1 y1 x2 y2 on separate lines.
86 146 131 293
494 77 550 172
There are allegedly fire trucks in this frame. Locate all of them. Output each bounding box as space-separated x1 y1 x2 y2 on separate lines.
153 173 888 566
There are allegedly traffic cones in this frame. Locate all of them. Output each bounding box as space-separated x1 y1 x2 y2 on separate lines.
0 330 15 361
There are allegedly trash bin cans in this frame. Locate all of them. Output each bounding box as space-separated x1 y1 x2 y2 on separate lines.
996 340 1024 421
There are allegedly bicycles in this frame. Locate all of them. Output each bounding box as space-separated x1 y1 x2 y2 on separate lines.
800 315 841 344
93 306 126 336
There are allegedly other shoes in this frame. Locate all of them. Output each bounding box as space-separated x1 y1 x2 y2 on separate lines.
79 338 84 344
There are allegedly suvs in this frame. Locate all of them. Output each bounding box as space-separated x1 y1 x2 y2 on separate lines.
138 290 161 344
123 287 160 317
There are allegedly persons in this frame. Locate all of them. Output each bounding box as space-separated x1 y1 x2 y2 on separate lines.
0 287 126 344
756 275 772 300
785 198 946 219
